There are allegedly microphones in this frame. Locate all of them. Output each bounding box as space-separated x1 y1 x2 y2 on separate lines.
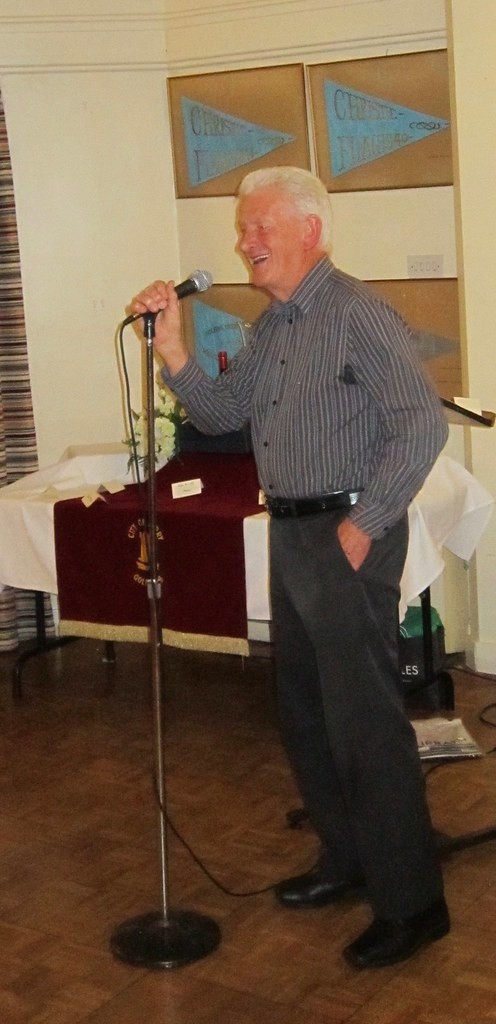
123 270 212 324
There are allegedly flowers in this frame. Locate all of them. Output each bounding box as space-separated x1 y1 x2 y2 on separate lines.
121 388 185 479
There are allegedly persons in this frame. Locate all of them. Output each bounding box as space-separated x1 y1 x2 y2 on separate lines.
124 168 450 967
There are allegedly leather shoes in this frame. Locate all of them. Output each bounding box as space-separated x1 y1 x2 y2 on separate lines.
343 902 453 970
273 860 370 911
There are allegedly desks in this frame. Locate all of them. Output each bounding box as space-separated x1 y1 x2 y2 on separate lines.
1 454 456 712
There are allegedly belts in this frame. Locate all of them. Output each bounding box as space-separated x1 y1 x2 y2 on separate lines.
263 489 369 520
